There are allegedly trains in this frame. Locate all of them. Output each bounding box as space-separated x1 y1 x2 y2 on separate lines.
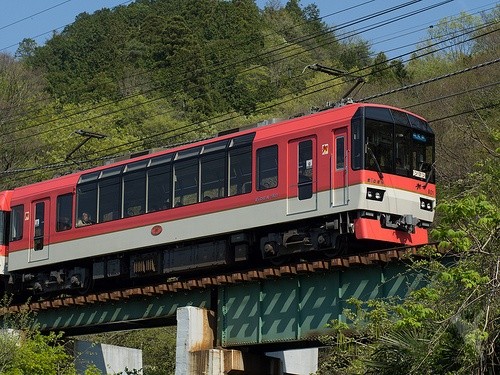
1 76 437 307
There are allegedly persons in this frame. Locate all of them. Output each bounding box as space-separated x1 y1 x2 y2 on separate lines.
125 195 214 221
76 211 92 228
